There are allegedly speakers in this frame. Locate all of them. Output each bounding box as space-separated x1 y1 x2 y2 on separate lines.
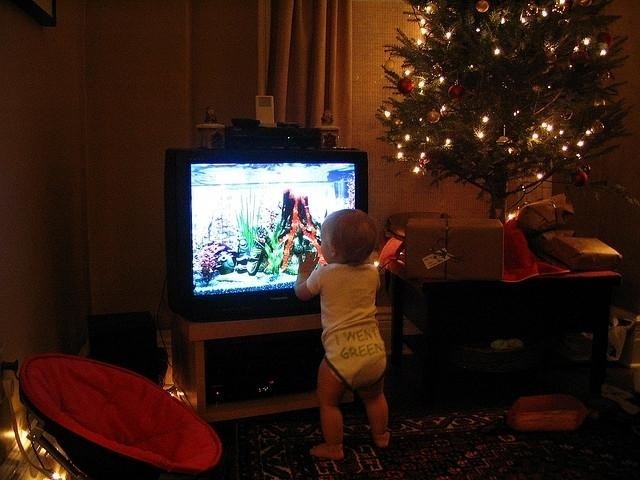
87 312 160 385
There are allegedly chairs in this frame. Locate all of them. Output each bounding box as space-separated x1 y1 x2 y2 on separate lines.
18 354 225 480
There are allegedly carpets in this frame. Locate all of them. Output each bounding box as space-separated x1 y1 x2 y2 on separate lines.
196 401 639 480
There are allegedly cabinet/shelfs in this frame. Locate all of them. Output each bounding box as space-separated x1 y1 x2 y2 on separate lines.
168 313 355 422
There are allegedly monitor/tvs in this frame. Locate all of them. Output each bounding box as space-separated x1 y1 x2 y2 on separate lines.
164 147 369 323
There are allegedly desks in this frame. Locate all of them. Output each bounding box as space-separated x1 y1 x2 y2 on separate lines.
385 268 621 405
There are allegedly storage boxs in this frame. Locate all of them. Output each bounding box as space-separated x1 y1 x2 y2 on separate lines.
406 219 504 283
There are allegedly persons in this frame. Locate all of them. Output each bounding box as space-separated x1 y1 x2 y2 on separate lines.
292 209 391 462
